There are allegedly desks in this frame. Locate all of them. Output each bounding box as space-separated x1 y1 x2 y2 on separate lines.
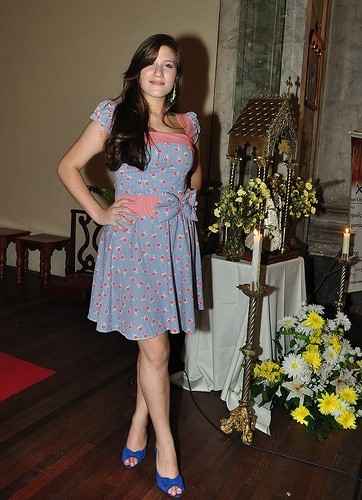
169 252 308 437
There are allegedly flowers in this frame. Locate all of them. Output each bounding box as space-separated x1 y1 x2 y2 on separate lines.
274 173 319 218
251 301 362 443
204 177 277 237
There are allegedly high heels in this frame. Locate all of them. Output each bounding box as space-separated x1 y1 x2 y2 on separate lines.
122 428 148 469
152 449 187 498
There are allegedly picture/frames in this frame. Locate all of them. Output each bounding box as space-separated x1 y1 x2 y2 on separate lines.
304 28 324 111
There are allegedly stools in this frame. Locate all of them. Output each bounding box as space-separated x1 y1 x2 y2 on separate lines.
0 228 31 280
15 233 75 289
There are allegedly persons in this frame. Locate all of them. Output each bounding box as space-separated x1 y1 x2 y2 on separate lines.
57 34 202 498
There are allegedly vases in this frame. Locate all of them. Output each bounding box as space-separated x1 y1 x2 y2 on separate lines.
223 224 246 262
281 222 299 251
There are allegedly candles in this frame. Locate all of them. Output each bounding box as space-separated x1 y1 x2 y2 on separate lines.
249 229 263 292
341 227 351 260
